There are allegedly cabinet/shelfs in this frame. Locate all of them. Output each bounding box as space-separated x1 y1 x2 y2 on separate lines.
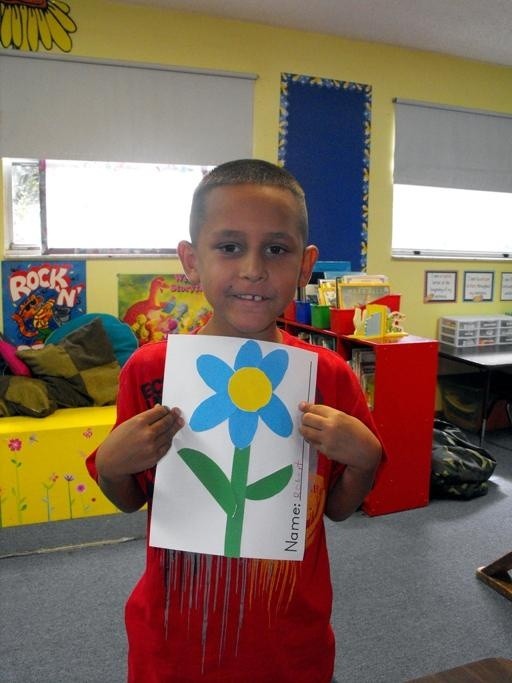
275 314 441 517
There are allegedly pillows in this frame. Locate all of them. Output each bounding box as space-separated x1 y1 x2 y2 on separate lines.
45 313 139 367
13 317 122 409
0 340 32 376
0 373 57 418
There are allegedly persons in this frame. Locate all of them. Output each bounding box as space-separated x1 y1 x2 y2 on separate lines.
83 157 391 681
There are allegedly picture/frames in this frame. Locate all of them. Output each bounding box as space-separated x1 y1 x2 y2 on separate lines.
463 270 494 301
425 271 457 302
500 271 512 301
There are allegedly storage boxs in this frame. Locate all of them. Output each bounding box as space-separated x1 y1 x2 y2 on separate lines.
284 293 402 335
437 316 512 347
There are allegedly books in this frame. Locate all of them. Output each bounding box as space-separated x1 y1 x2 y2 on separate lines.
287 331 378 411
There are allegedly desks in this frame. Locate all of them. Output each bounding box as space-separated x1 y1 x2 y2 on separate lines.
438 342 512 448
